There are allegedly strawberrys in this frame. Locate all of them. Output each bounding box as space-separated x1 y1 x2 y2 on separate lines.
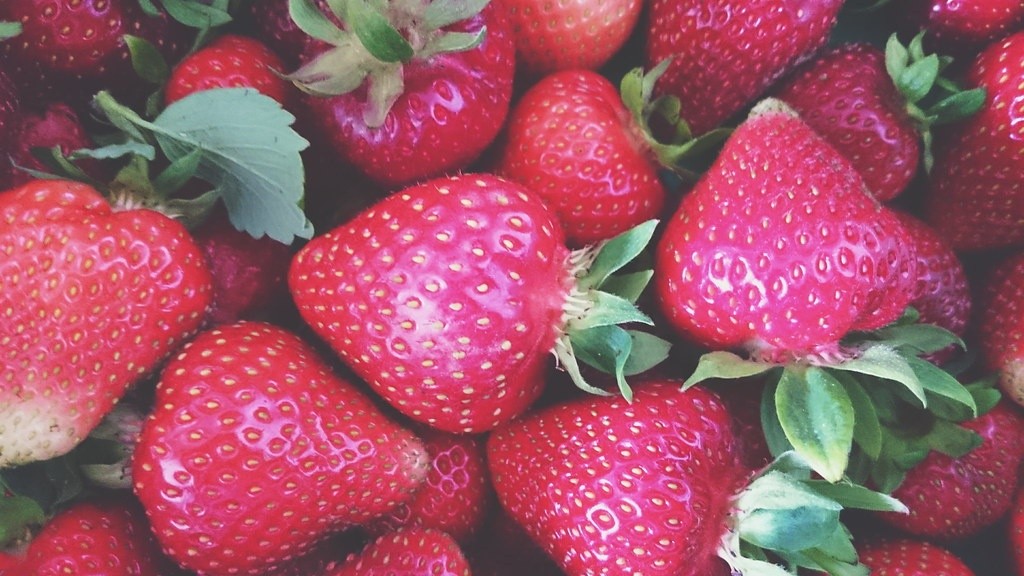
0 0 1024 576
